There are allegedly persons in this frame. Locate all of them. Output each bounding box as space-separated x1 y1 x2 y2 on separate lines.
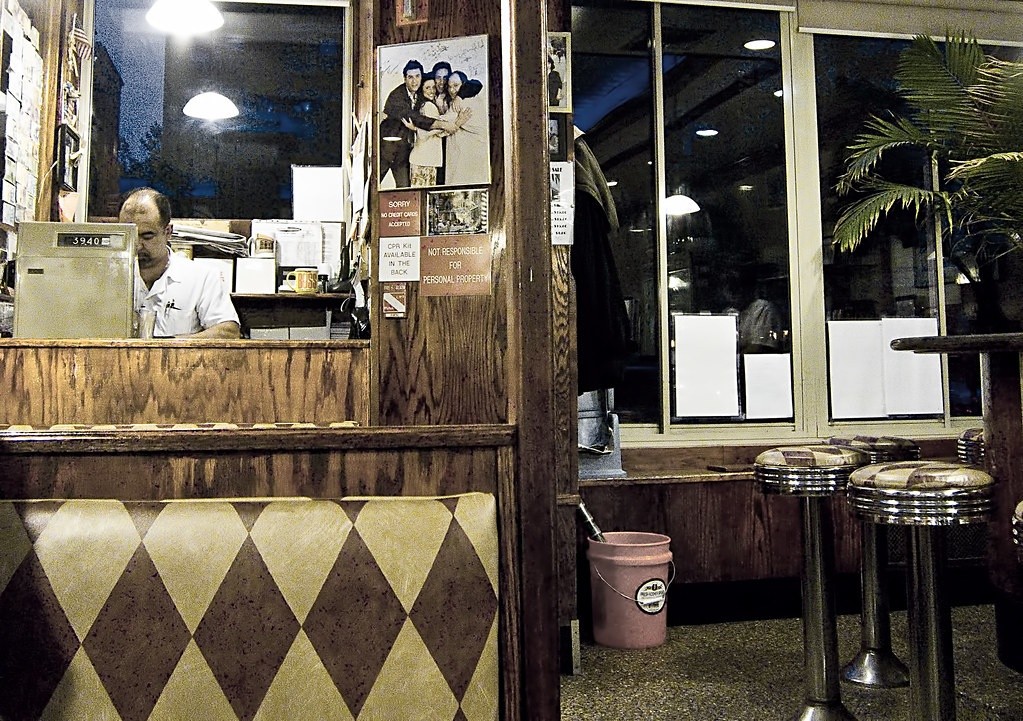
118 188 241 339
548 55 563 105
408 72 472 187
431 60 483 184
379 59 460 188
401 70 485 184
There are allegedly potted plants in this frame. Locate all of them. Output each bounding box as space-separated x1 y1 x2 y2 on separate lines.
943 238 1021 334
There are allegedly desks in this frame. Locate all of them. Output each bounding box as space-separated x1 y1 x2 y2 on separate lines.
229 291 356 340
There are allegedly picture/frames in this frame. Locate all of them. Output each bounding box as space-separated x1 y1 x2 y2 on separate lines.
546 31 572 114
549 112 567 162
550 161 575 246
376 33 493 191
57 123 81 193
426 188 489 236
668 309 746 422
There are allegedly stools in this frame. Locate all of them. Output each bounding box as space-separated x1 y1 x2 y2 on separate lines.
843 460 998 721
753 443 871 721
828 436 922 688
956 427 987 465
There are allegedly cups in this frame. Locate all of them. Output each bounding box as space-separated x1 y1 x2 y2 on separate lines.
245 231 277 259
285 267 321 295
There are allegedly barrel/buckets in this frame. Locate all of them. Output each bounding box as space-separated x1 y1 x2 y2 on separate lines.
583 527 675 649
